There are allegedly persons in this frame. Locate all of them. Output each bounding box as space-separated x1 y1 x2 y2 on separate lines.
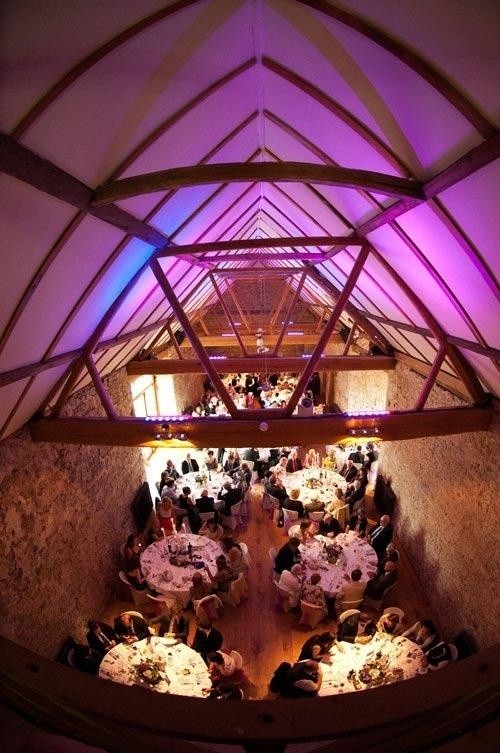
120 444 263 612
72 606 246 702
258 444 401 623
188 374 341 417
269 613 451 702
